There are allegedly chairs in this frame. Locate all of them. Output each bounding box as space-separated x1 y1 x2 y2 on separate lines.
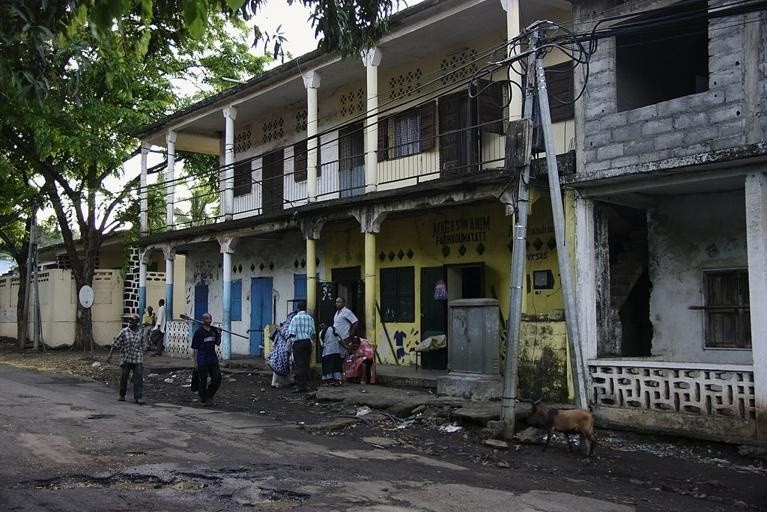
410 331 443 372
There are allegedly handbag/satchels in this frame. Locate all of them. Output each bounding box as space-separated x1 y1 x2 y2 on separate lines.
190 365 205 391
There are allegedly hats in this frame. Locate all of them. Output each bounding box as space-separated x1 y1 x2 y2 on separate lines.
130 314 140 320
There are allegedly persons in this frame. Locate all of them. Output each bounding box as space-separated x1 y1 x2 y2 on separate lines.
110 314 143 404
141 306 156 350
265 295 375 390
149 299 167 355
190 313 221 407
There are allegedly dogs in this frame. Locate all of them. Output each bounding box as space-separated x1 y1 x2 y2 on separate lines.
528 399 595 458
